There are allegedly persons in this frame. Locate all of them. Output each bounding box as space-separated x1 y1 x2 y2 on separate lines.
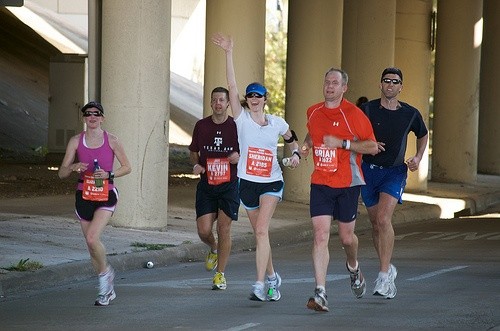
188 86 240 290
358 68 427 299
298 67 378 312
210 30 301 301
357 96 368 107
58 101 132 307
292 150 301 159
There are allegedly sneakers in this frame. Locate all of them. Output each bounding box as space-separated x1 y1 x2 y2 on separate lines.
306 288 329 313
249 283 266 301
212 272 227 290
373 270 392 296
94 289 116 306
383 264 398 299
346 259 366 298
96 269 115 297
205 249 219 272
265 272 281 302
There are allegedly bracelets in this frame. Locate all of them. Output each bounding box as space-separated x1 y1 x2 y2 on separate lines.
345 139 350 149
193 164 198 168
108 171 114 178
342 139 347 148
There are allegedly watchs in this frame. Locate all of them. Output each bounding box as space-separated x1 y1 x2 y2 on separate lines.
301 146 309 151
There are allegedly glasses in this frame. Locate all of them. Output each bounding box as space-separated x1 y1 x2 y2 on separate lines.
381 78 403 85
82 110 102 117
246 92 263 98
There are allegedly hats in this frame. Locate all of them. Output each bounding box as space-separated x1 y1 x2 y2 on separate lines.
245 83 266 96
80 101 103 114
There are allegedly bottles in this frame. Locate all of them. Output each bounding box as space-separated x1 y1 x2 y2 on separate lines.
94 158 103 187
283 158 296 170
